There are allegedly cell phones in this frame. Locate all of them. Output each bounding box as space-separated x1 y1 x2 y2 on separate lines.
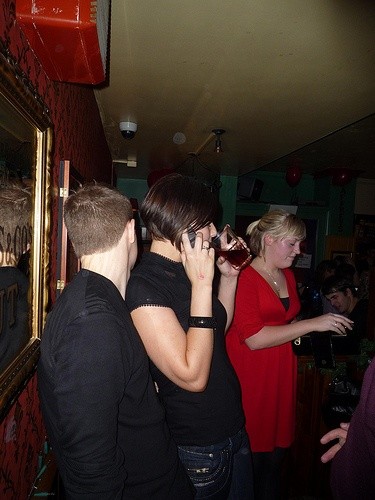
179 229 197 248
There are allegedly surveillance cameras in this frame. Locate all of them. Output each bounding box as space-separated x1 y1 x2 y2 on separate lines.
120 121 138 139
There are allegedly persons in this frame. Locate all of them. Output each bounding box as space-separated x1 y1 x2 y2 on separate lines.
304 255 369 369
40 185 197 500
126 172 255 500
320 357 375 500
226 208 354 500
0 188 32 368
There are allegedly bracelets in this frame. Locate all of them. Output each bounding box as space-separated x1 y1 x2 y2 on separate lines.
189 316 215 329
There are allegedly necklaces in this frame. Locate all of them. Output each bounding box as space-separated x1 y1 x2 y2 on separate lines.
269 274 276 285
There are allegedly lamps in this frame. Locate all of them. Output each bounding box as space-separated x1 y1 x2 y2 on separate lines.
212 128 225 153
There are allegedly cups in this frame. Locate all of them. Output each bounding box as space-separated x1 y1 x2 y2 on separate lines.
210 226 252 271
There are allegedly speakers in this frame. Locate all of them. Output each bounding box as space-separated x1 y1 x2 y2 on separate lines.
237 176 264 202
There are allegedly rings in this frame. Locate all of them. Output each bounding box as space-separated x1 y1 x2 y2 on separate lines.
202 246 209 250
335 322 337 325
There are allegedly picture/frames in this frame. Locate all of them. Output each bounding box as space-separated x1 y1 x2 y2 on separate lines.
0 44 51 424
60 160 87 291
